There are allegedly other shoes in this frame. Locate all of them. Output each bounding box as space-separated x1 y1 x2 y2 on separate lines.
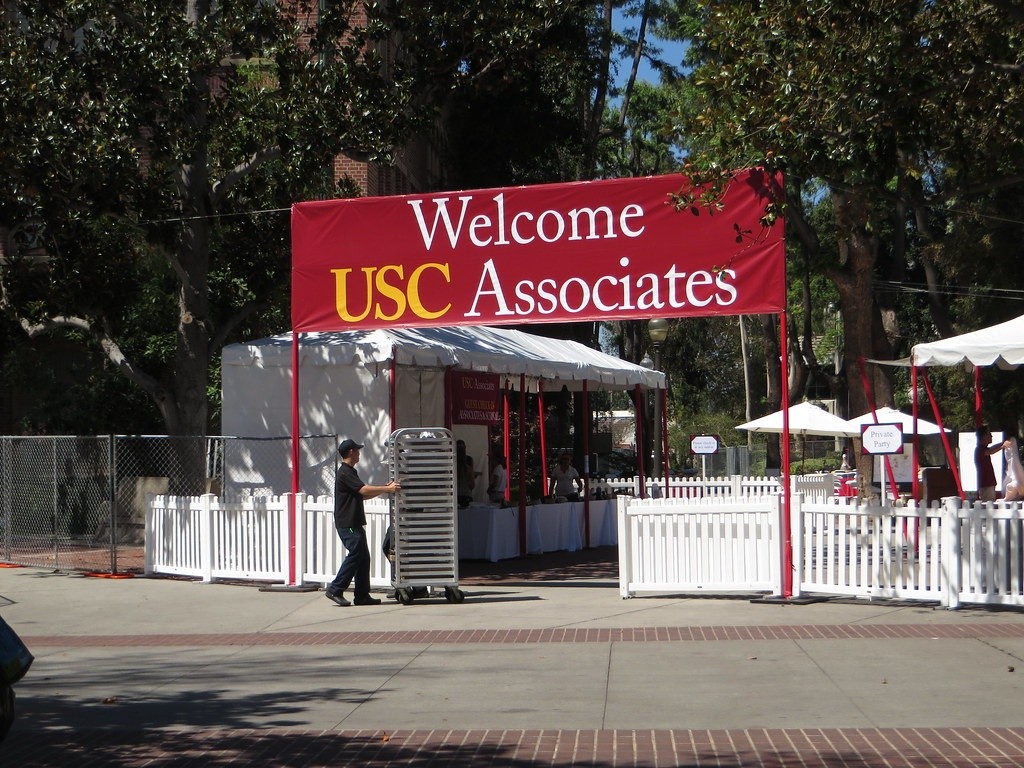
414 592 429 598
354 596 381 605
325 591 351 606
387 592 395 598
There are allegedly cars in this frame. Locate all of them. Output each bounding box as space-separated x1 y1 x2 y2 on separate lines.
829 468 858 504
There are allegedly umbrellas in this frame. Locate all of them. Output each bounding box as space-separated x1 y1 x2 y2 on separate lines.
734 402 951 475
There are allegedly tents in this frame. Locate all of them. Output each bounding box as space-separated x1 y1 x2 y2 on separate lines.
859 315 1024 559
222 323 668 561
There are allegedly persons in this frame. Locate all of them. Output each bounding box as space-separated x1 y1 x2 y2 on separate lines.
974 418 1024 502
487 449 507 502
326 439 402 606
383 438 476 598
620 465 639 480
840 446 852 469
681 458 698 481
549 454 582 501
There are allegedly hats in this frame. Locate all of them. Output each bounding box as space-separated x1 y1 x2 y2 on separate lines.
339 439 364 457
560 455 573 460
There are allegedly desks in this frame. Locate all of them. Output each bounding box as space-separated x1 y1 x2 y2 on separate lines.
458 498 619 563
